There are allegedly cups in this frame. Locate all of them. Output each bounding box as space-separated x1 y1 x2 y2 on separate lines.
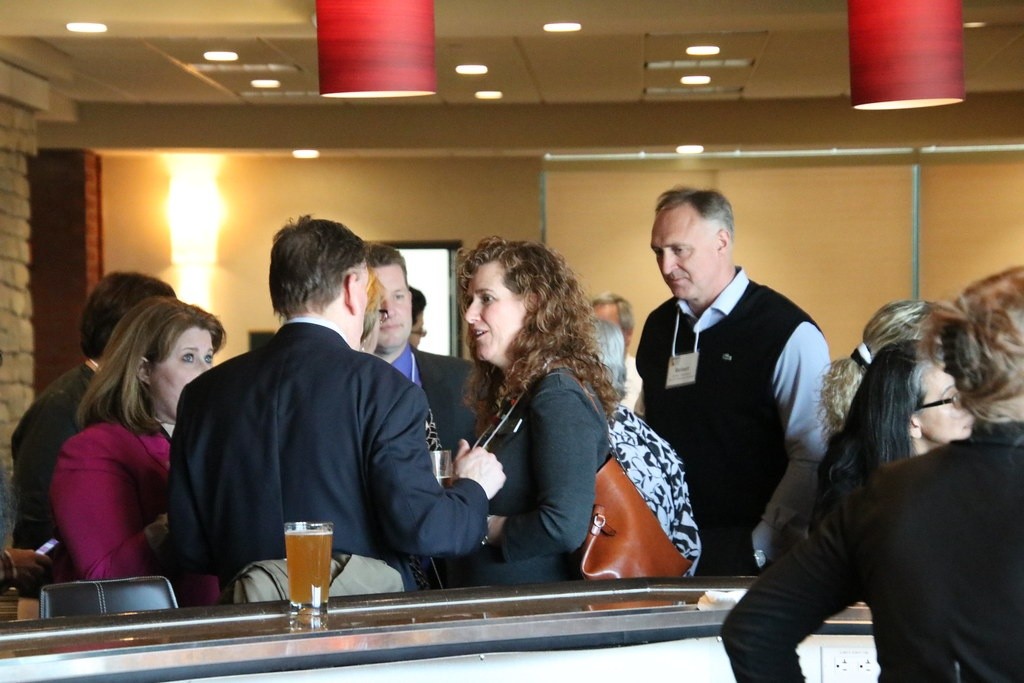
430 450 453 490
284 521 334 615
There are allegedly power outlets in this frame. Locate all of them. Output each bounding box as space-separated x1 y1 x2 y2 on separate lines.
820 647 880 683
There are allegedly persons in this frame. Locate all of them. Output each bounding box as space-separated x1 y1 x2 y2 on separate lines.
46 295 228 608
452 235 624 589
633 186 832 578
721 265 1024 682
166 215 507 608
0 241 1024 683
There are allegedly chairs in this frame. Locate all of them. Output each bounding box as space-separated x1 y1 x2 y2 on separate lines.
233 550 405 603
39 575 179 619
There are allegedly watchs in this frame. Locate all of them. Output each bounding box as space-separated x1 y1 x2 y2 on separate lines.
753 550 767 567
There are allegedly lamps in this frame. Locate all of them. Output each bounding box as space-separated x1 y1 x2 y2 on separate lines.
846 0 967 111
314 0 438 99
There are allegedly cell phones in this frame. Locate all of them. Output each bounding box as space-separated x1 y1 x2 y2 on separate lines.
36 538 60 555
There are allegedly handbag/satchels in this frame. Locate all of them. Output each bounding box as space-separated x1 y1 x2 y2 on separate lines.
577 455 694 611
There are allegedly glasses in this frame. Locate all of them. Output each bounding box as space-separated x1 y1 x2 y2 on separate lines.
914 392 962 411
411 330 427 337
378 309 389 324
